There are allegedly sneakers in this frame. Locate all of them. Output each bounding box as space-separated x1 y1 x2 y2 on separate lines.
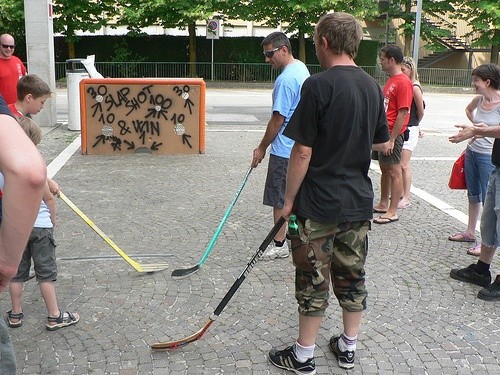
450 263 492 287
268 344 317 375
257 238 289 260
397 199 410 208
477 275 500 301
329 335 356 369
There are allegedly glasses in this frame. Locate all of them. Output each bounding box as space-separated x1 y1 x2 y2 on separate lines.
262 45 288 58
0 44 16 48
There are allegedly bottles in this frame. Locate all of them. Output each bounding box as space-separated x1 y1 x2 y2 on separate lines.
288 215 300 237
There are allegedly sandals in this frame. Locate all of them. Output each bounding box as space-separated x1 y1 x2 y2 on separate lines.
5 308 24 328
46 310 80 331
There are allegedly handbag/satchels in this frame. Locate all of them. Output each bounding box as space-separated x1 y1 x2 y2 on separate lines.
448 149 468 190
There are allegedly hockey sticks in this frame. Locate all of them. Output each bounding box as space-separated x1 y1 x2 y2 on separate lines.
55 188 168 273
150 215 285 352
170 164 255 279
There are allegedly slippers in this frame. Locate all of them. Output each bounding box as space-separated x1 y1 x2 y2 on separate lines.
448 232 476 242
373 207 387 213
373 216 399 224
467 244 481 256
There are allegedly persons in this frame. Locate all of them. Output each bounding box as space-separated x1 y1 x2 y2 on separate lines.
448 63 500 257
0 74 60 233
0 91 48 375
449 122 500 302
390 56 425 209
369 45 413 224
0 34 28 104
251 33 311 260
269 12 391 375
7 116 79 331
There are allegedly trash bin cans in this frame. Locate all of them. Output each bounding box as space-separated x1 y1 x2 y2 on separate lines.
65 58 90 131
